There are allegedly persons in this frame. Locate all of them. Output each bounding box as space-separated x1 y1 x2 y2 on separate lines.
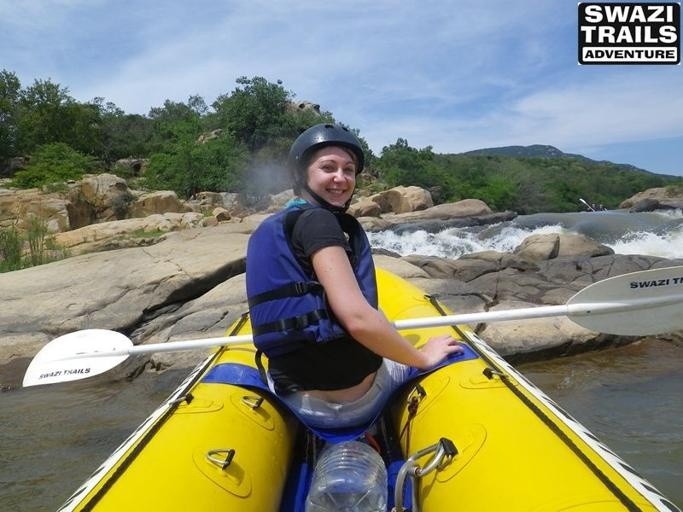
587 203 606 212
245 123 465 430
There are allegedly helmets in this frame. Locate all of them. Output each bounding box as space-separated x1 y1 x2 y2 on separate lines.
287 123 364 194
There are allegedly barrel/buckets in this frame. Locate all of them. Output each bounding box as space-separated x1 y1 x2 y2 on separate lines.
302 441 388 512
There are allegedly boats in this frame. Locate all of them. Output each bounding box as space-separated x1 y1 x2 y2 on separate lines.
48 263 683 511
580 211 609 215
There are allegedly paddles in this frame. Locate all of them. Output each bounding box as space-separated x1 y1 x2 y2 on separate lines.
22 265 683 387
